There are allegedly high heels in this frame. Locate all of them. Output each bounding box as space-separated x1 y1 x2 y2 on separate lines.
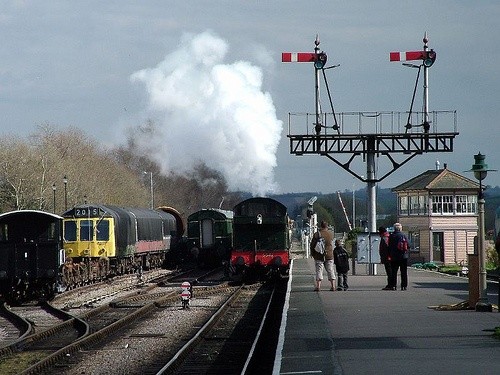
386 285 397 290
401 286 407 290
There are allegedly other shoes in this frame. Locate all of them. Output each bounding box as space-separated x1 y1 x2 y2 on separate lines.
337 287 342 291
330 286 336 291
344 285 349 288
314 287 319 291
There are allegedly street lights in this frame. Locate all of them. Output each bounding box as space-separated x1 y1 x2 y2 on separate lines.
142 171 154 210
63 174 68 212
461 151 499 312
52 182 57 214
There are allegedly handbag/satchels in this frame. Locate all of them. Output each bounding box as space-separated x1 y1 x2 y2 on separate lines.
398 233 408 252
314 230 324 255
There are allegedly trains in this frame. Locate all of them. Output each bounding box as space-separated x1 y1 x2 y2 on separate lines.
228 196 293 279
62 203 186 278
183 208 234 269
0 210 66 307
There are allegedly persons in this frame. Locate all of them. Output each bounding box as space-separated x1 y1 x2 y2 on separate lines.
379 223 411 291
311 220 336 292
333 239 350 291
